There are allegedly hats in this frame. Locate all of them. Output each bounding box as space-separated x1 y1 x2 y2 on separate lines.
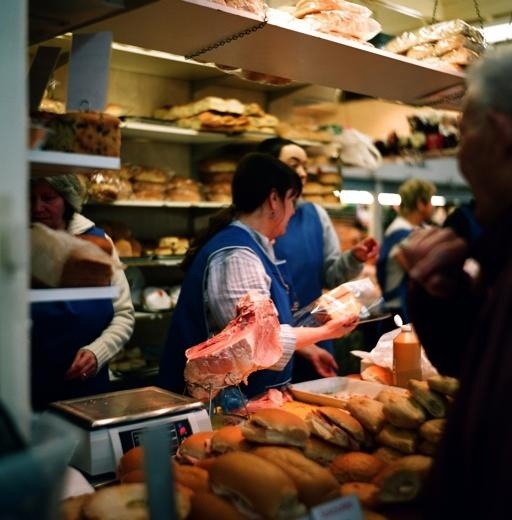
42 174 83 212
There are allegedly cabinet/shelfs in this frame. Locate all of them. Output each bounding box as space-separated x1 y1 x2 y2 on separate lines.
27 118 345 322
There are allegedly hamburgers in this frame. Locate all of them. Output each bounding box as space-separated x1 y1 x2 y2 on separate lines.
60 375 461 518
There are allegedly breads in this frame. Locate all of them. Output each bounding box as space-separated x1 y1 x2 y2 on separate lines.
382 19 486 69
108 344 160 374
63 94 344 256
208 0 266 20
141 284 183 312
310 286 361 325
294 0 373 46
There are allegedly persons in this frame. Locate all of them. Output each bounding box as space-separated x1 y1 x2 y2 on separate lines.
376 176 437 331
394 42 512 518
154 154 364 414
248 137 379 379
30 169 136 405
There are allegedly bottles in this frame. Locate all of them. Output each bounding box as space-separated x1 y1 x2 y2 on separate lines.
391 313 423 386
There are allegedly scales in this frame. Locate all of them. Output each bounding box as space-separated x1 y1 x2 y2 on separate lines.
41 386 212 483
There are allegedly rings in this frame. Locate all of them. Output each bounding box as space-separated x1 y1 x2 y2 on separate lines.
84 373 86 376
398 239 409 250
